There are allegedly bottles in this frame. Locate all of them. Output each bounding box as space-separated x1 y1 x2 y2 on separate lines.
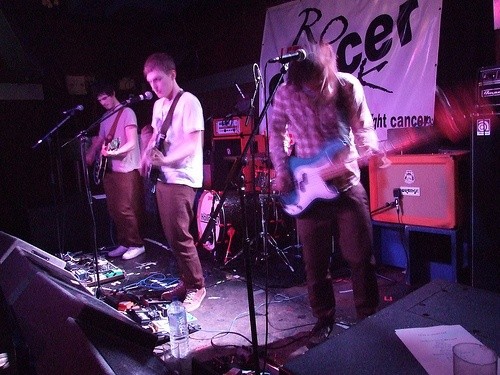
167 296 188 358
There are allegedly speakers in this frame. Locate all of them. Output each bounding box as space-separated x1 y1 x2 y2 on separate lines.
470 111 500 293
0 231 181 375
369 149 473 229
212 135 267 193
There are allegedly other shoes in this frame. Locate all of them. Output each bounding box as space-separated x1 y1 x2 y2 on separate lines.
122 246 145 260
308 318 333 346
108 245 128 257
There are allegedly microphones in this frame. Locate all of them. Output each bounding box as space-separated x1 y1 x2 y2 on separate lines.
392 188 401 213
63 105 84 114
256 64 264 88
121 91 153 104
233 83 246 99
267 49 306 63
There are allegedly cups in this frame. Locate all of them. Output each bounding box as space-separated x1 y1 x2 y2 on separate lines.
453 343 497 375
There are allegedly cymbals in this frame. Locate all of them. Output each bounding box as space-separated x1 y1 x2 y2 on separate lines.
225 155 269 162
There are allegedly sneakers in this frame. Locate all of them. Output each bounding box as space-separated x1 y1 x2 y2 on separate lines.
161 281 186 300
182 287 206 312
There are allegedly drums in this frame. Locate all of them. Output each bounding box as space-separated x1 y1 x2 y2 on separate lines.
196 189 247 253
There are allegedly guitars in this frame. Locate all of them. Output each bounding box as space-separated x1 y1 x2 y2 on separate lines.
147 133 165 197
93 137 120 185
279 131 430 217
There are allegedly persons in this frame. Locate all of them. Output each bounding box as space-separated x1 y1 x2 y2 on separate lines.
140 52 207 314
270 43 378 344
86 86 145 260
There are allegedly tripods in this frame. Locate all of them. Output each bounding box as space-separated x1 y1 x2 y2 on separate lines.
222 177 298 279
